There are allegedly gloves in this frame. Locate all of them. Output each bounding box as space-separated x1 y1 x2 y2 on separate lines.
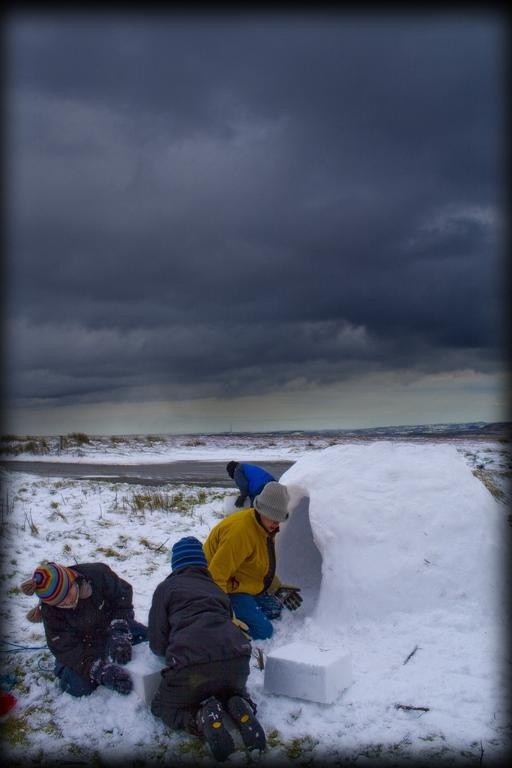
233 494 247 508
92 662 134 695
229 614 252 642
274 582 304 612
106 627 133 664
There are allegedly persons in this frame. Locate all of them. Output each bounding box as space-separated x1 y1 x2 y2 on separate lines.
147 536 266 762
226 461 276 508
201 481 304 671
18 559 149 697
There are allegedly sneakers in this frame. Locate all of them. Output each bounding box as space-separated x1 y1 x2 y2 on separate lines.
196 698 236 762
226 694 268 753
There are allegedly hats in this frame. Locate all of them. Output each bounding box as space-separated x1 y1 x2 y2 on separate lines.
171 536 209 571
251 479 291 523
21 562 81 611
225 460 239 479
